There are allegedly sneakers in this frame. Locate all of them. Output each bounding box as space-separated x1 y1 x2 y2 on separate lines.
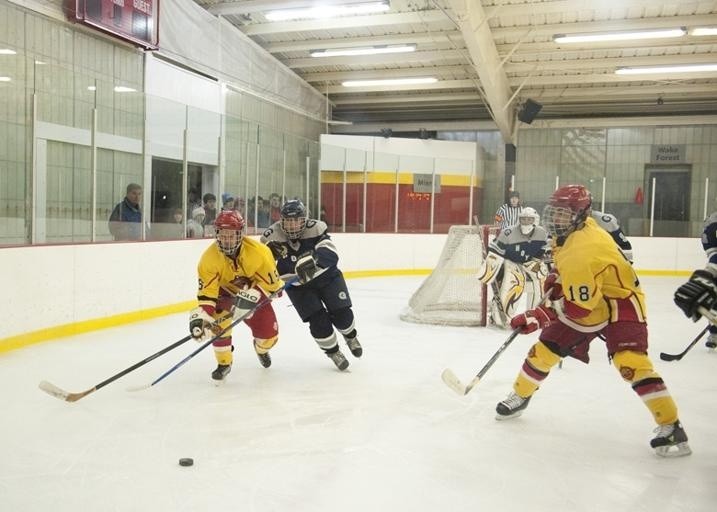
328 336 362 369
212 365 231 379
706 333 717 347
496 393 532 415
651 421 688 447
259 353 270 368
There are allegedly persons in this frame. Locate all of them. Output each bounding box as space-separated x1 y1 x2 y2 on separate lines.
495 190 523 230
260 198 363 372
189 209 286 381
495 183 688 448
673 209 717 348
476 206 553 330
522 208 634 273
108 183 330 242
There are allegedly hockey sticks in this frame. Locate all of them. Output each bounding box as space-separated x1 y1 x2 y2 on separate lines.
660 324 711 361
473 215 514 330
441 286 553 397
39 275 300 403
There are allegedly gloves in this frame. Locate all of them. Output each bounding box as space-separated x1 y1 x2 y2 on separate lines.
294 252 316 284
190 308 204 337
510 269 563 334
674 270 717 322
231 288 261 319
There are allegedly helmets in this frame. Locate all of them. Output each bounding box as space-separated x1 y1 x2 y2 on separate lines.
543 185 592 237
215 211 245 255
281 200 306 238
519 207 540 234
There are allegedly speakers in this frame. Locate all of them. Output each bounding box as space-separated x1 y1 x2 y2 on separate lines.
518 98 542 126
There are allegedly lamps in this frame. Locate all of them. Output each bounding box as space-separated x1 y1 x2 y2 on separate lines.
552 24 717 76
264 0 439 87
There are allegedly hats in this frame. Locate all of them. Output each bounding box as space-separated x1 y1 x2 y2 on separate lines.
190 205 205 218
222 193 234 204
